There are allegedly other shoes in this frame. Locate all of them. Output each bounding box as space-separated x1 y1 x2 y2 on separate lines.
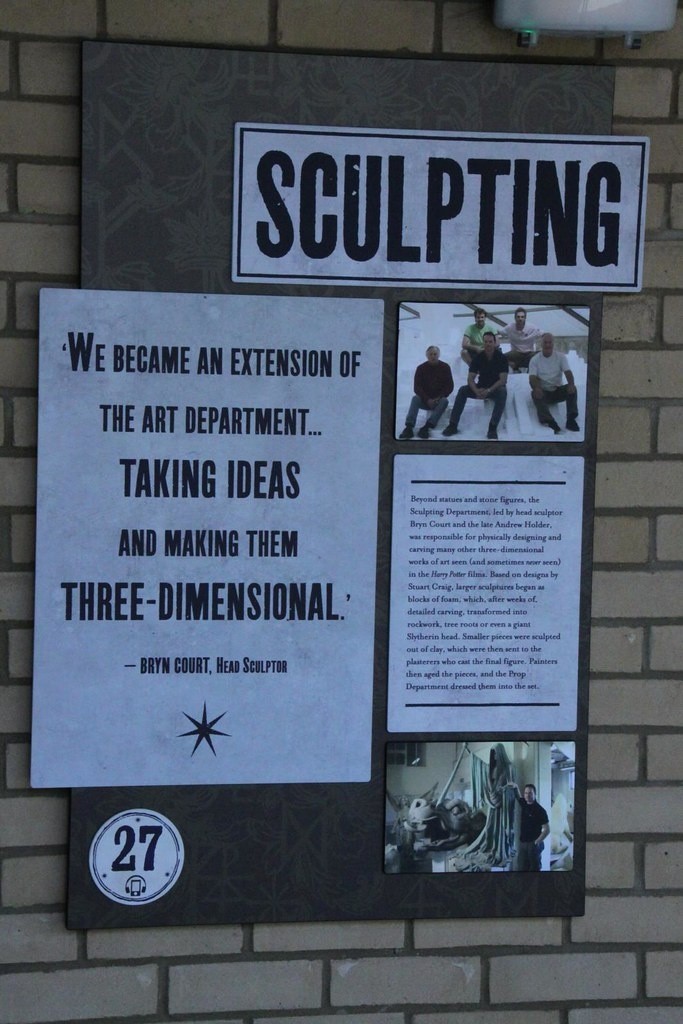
418 426 429 438
400 428 414 439
442 426 457 436
549 421 560 433
565 420 580 431
488 431 497 440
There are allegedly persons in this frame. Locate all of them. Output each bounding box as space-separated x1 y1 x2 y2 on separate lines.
398 345 454 438
508 782 550 870
442 307 580 439
